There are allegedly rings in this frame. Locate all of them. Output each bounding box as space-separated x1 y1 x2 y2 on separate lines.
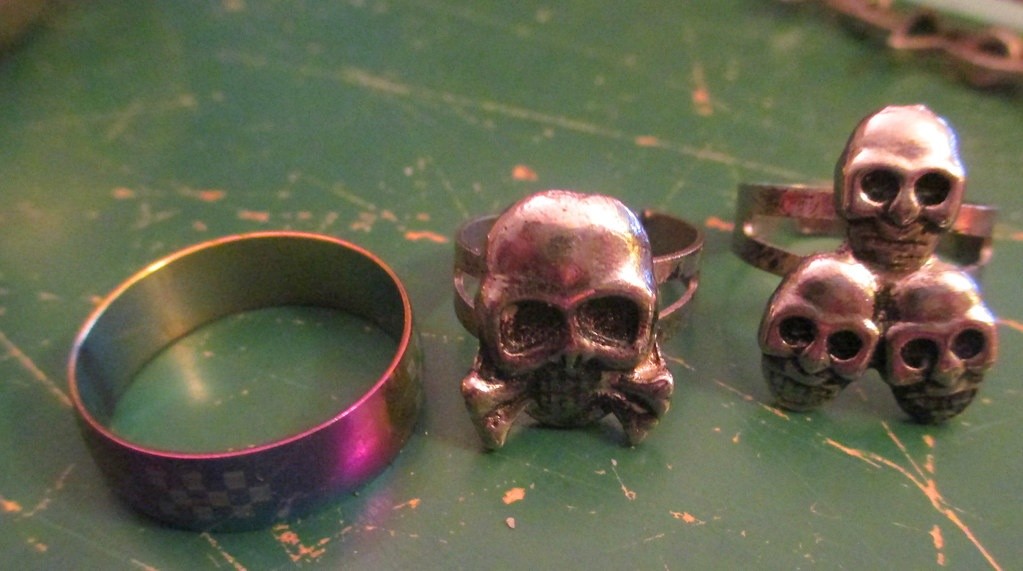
67 232 425 532
733 105 997 426
454 191 704 452
